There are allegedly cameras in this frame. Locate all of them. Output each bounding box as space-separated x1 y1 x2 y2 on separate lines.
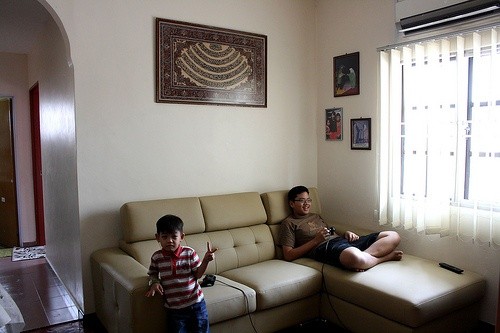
326 227 336 236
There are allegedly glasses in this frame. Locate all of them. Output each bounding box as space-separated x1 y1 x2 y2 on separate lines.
293 199 312 203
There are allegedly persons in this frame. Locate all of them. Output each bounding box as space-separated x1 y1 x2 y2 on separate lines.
278 185 403 272
146 214 217 333
326 111 368 144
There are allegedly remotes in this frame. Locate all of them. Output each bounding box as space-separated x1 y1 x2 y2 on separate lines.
439 262 464 275
